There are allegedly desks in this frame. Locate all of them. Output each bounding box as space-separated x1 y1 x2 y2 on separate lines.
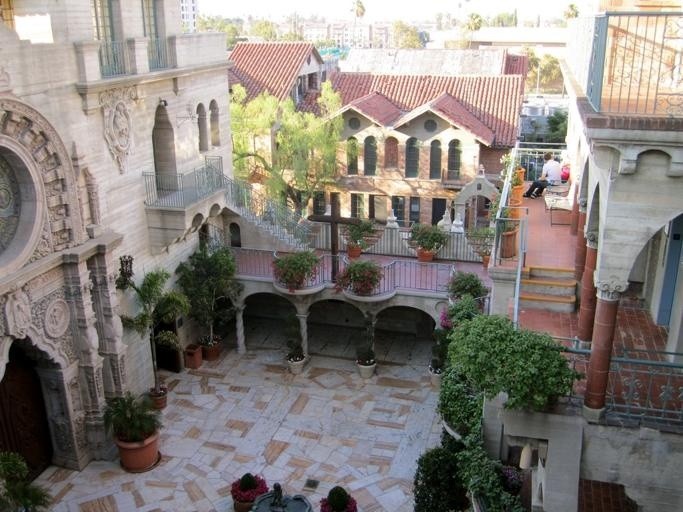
547 186 568 196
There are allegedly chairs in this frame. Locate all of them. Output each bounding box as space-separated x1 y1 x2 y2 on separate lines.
544 181 575 227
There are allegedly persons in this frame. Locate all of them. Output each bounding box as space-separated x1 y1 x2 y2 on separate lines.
9 293 27 330
522 153 562 200
113 104 130 146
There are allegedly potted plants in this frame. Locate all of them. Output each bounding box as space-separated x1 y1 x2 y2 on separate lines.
174 245 243 361
487 152 525 258
346 219 374 257
467 228 494 267
102 391 160 473
284 311 306 375
428 329 449 387
118 269 191 411
350 329 377 379
407 223 449 262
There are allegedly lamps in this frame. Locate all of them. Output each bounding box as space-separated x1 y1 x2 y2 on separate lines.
517 441 534 472
115 255 133 292
